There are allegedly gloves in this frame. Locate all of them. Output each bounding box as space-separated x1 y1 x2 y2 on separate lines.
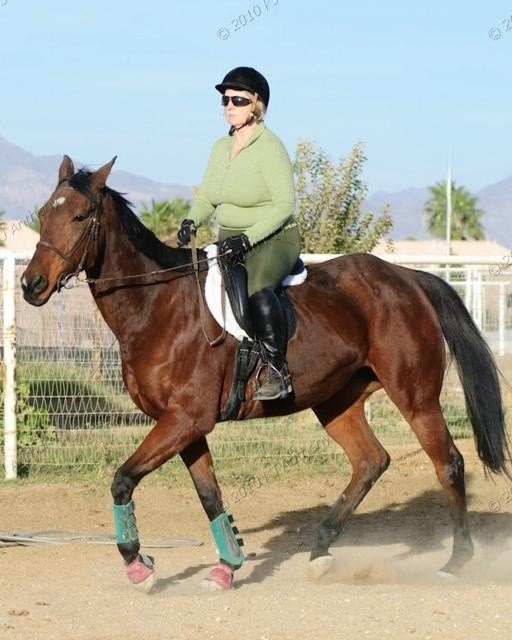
217 233 251 263
175 219 198 246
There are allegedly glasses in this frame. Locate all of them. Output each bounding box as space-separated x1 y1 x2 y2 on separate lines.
214 66 270 108
221 95 253 106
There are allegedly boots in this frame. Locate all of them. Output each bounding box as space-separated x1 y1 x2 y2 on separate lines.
248 286 294 402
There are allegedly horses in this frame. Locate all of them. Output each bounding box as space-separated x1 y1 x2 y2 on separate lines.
20 154 512 595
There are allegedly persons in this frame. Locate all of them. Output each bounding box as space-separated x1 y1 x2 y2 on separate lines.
176 65 305 404
